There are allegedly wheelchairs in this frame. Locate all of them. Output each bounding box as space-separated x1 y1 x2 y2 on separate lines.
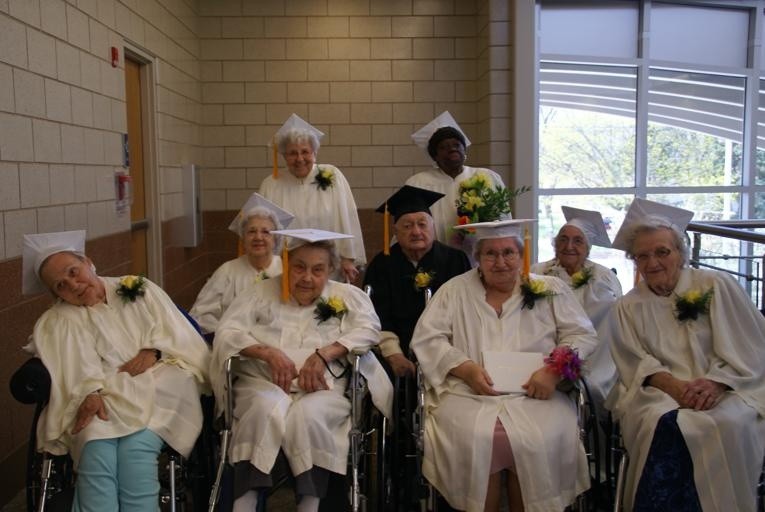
418 288 599 510
598 409 765 512
207 285 387 508
16 362 187 512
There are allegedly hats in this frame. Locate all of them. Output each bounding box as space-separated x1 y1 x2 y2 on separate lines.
229 193 292 257
22 230 86 293
376 186 446 255
268 114 324 178
613 198 694 285
456 219 536 280
270 228 356 304
562 206 612 249
411 110 472 166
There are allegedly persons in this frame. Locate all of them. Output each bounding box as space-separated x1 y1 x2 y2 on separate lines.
530 205 622 324
410 219 598 512
209 228 381 512
404 110 513 272
605 198 765 512
188 192 282 343
363 186 471 375
21 231 213 512
257 114 367 283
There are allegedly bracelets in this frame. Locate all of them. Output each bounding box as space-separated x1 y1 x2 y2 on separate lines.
154 349 162 360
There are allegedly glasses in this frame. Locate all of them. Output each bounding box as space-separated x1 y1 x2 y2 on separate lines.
476 247 521 257
316 348 350 379
632 246 680 260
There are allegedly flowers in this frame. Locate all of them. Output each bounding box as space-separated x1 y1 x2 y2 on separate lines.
454 171 535 240
542 339 591 388
